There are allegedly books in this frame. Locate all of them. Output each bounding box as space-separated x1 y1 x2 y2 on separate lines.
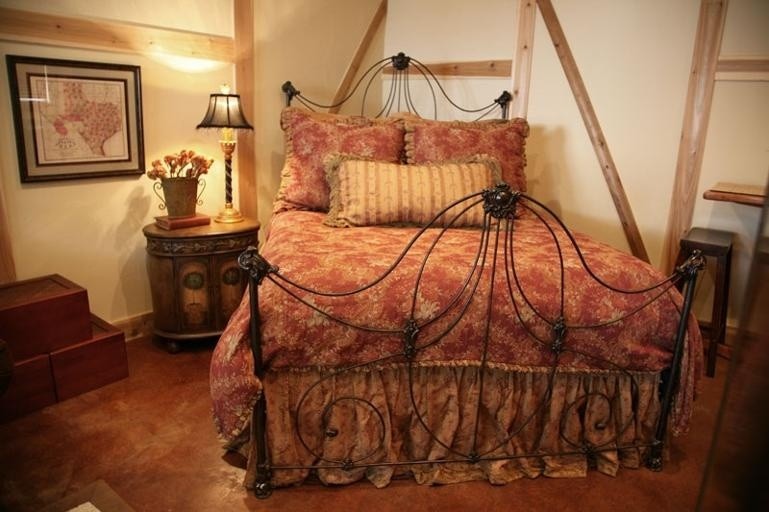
154 212 209 230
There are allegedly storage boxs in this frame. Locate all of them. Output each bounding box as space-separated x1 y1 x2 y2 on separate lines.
0 273 129 421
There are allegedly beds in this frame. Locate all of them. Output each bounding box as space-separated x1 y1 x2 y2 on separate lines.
238 52 705 499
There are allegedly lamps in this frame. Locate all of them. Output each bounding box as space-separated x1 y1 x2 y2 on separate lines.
197 83 254 224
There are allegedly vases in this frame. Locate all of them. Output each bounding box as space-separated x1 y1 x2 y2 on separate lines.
154 178 206 219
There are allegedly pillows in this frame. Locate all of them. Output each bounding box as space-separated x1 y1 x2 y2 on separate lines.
399 110 530 218
321 152 513 232
272 107 404 213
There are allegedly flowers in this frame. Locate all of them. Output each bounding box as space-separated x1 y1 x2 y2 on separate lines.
145 150 212 180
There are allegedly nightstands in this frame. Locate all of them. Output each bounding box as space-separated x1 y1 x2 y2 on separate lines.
142 218 260 354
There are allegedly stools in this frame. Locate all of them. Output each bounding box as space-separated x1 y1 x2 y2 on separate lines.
673 227 736 379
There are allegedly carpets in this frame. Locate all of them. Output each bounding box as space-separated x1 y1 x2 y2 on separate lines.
34 480 137 512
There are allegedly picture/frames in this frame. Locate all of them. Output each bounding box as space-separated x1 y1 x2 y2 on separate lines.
5 55 145 182
